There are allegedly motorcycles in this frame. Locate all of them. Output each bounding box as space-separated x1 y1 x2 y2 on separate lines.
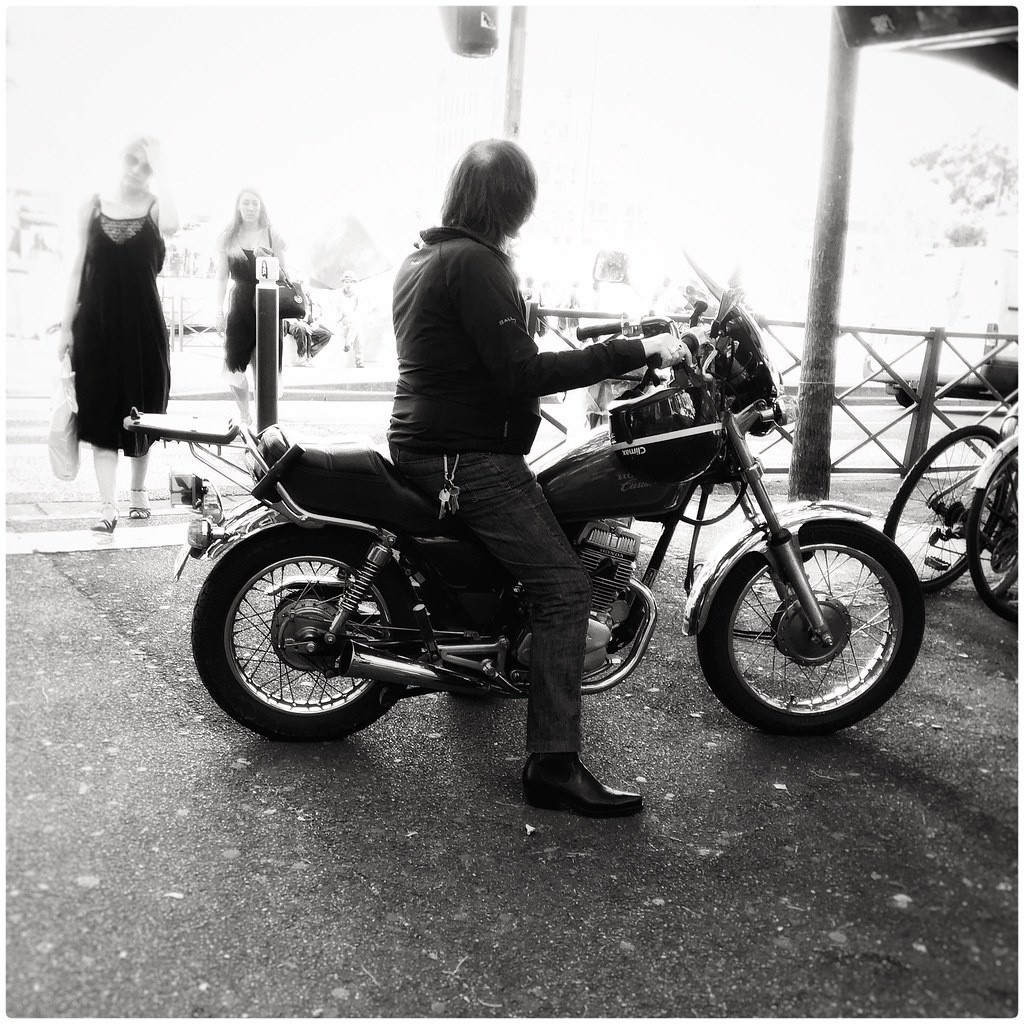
124 249 926 744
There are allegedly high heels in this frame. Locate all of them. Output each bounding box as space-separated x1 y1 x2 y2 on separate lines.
129 489 151 519
90 502 120 534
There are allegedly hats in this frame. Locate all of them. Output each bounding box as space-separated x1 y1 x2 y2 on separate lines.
339 276 357 284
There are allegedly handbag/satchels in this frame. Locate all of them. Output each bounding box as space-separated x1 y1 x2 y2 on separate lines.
276 267 306 320
48 352 81 482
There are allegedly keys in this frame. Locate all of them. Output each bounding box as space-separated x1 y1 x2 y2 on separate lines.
438 482 460 519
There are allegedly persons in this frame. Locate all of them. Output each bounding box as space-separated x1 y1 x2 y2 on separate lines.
388 138 690 813
57 134 180 533
213 188 288 425
336 271 372 368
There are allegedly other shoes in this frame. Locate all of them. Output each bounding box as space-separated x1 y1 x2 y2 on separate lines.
356 363 365 368
344 345 350 352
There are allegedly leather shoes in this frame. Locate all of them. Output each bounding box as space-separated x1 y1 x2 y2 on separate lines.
521 752 644 820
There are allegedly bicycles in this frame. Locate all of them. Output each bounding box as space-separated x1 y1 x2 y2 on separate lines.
884 402 1018 624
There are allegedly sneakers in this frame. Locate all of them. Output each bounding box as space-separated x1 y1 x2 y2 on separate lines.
293 356 314 368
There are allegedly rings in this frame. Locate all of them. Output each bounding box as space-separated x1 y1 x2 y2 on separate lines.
670 344 682 355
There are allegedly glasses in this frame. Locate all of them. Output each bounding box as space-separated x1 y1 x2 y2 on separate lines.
345 280 353 283
123 154 151 176
